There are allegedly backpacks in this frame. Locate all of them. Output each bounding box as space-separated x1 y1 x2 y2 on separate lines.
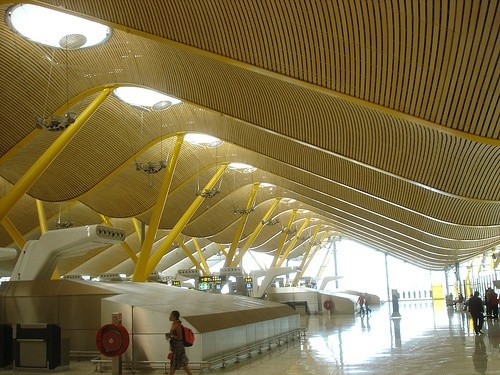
183 324 194 347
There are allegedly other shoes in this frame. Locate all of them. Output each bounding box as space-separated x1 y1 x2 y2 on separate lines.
475 328 483 334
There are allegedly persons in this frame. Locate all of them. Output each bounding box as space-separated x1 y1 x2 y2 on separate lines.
391 289 400 316
358 293 365 314
469 292 485 334
365 293 371 314
164 310 193 375
449 288 500 320
